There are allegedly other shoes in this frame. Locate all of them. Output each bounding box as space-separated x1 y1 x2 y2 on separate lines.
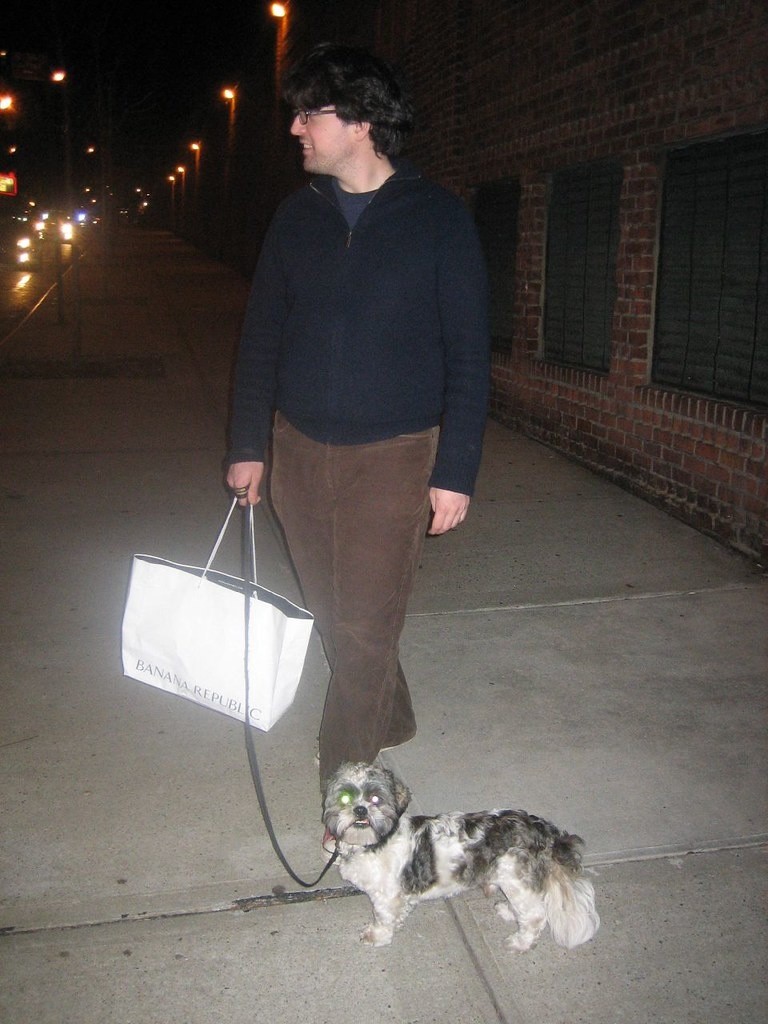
316 729 419 767
321 827 343 865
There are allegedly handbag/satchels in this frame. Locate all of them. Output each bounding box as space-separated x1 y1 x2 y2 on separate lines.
120 497 314 732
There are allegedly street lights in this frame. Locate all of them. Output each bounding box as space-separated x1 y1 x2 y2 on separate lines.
52 68 78 217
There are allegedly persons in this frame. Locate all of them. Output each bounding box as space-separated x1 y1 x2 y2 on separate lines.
226 40 492 866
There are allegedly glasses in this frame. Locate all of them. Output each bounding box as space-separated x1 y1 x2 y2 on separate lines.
292 108 336 124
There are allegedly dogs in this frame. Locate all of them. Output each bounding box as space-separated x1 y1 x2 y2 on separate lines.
321 761 601 951
